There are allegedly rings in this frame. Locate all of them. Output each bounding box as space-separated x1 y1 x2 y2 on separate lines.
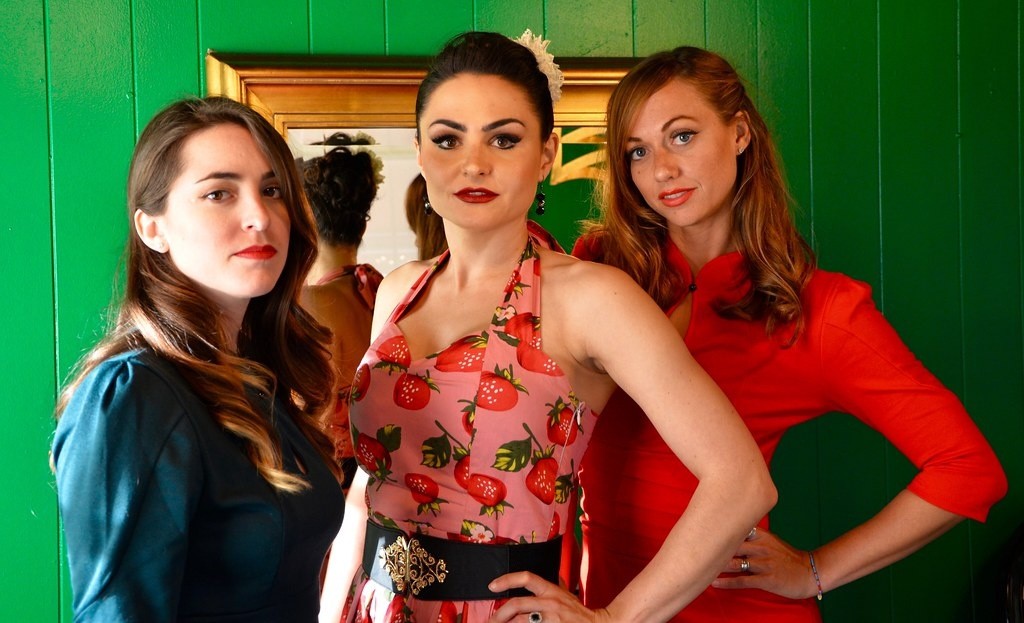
741 558 750 572
747 525 756 540
530 612 541 623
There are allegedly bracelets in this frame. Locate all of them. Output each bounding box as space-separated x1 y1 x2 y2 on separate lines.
807 549 824 601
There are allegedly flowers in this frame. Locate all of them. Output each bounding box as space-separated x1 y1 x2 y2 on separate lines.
510 29 565 103
349 132 384 193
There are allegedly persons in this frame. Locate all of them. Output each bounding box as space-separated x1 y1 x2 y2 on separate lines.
570 47 1007 623
320 31 777 623
297 132 385 622
405 172 448 261
53 97 346 622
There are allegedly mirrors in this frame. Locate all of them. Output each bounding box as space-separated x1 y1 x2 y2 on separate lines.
205 51 650 593
